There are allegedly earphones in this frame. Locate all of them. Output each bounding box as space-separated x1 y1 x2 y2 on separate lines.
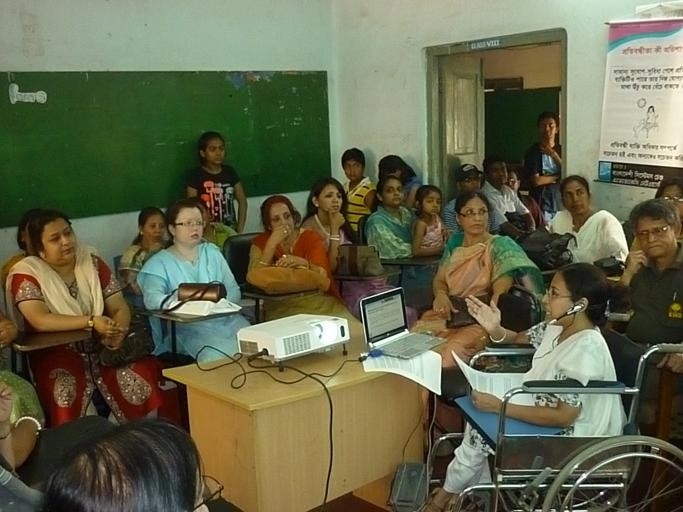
567 304 584 315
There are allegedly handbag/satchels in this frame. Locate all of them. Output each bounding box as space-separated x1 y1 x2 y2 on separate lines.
446 295 490 328
336 244 384 276
102 316 156 367
506 211 525 229
518 229 572 270
178 283 227 301
246 261 330 295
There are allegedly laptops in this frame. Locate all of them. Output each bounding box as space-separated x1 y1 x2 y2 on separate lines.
359 287 448 359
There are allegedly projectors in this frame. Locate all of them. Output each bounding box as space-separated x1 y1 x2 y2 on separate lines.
236 313 351 372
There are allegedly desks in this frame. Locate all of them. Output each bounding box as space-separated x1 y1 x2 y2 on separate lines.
244 289 319 323
162 326 442 512
151 311 234 351
12 330 90 353
1 253 91 373
332 274 384 299
381 256 442 287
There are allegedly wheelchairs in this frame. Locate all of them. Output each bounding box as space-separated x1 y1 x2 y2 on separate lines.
386 309 683 512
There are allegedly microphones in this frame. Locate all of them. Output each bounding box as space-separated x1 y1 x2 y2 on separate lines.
549 313 567 325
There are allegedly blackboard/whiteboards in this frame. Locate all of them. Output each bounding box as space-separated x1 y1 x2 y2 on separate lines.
0 70 332 229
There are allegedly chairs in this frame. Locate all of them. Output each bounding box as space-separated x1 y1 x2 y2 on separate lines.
115 255 148 306
224 233 265 285
358 215 370 246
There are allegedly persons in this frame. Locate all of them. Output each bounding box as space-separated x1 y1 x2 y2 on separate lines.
523 111 562 215
185 132 247 233
0 147 683 511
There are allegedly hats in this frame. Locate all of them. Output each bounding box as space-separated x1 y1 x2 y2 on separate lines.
454 163 484 181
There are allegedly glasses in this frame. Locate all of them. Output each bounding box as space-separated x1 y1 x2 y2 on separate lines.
636 224 669 236
193 474 224 511
174 219 206 228
663 195 683 202
456 207 487 217
